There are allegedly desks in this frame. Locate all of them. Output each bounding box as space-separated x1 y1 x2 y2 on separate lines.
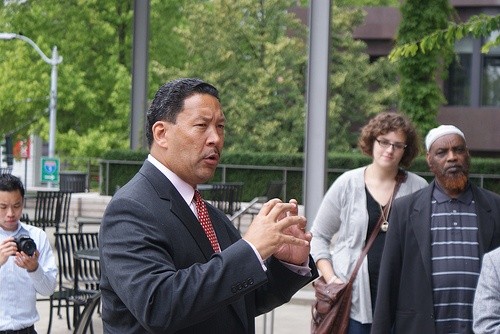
72 248 101 325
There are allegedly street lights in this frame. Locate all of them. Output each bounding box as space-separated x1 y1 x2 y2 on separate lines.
1 33 63 188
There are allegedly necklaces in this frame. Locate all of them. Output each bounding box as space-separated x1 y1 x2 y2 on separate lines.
374 185 395 231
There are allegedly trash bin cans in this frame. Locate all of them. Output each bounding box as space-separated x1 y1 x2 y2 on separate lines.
59 173 88 193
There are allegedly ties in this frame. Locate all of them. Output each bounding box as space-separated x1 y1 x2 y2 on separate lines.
193 190 220 255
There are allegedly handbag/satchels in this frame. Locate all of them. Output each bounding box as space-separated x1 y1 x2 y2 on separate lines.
310 277 353 334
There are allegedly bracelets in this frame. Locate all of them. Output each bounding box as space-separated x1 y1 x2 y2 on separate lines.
327 276 337 284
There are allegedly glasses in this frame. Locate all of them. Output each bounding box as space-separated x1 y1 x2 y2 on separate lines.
374 137 408 151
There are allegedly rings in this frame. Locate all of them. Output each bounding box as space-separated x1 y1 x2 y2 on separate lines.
297 223 306 229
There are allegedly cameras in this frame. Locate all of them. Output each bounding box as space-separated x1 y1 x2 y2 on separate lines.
11 235 36 256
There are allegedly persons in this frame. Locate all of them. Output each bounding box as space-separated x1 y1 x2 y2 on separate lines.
371 125 500 334
308 112 429 334
98 77 320 334
472 247 500 334
0 173 58 334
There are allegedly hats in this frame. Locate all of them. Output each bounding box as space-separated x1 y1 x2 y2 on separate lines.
425 125 467 153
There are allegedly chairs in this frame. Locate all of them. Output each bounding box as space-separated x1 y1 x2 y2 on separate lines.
27 191 72 233
210 183 241 215
47 233 101 334
